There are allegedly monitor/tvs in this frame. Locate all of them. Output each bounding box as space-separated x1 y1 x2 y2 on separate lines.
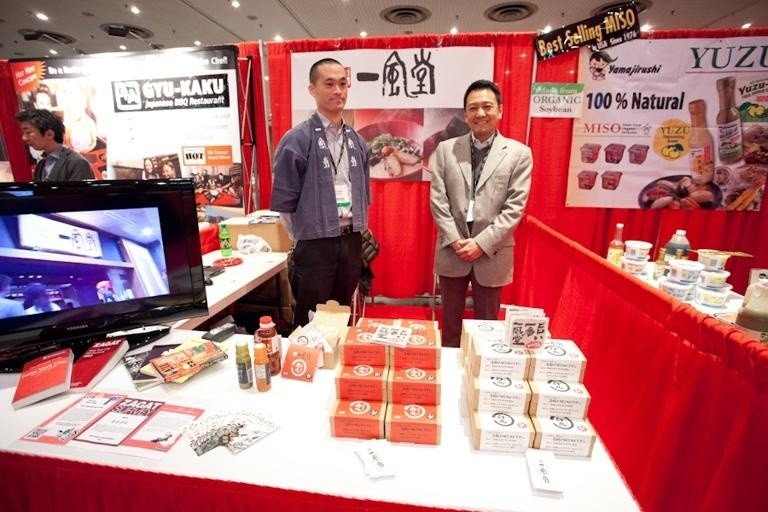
0 177 210 375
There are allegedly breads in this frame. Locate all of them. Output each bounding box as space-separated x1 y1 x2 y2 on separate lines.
743 126 768 165
642 177 715 210
722 164 767 211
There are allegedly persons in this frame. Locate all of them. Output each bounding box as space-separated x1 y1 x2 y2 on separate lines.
589 50 611 81
274 58 371 328
429 80 534 347
139 155 244 207
16 110 97 182
0 267 129 325
24 85 101 163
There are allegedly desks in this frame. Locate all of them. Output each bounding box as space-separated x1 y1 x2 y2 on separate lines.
148 249 291 332
0 330 642 511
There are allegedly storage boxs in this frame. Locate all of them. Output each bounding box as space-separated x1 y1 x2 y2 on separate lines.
215 208 294 253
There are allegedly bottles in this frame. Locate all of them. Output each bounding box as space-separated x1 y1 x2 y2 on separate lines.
686 99 715 185
219 225 231 257
715 76 743 164
235 315 282 393
653 229 691 279
607 223 626 270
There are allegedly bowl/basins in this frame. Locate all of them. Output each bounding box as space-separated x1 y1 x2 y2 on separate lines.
621 240 733 307
639 175 723 209
356 119 432 179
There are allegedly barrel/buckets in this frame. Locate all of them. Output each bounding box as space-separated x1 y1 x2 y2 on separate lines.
664 230 690 274
738 280 767 344
